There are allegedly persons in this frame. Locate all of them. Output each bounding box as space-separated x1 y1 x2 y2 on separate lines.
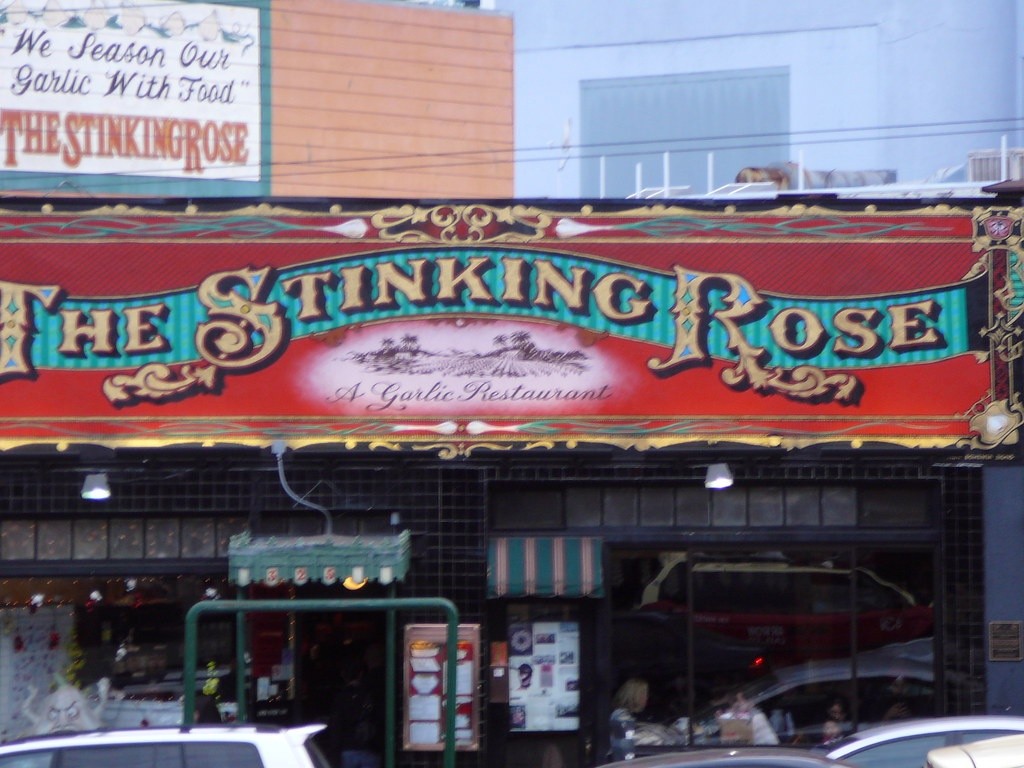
875 678 928 721
822 690 855 743
608 679 648 761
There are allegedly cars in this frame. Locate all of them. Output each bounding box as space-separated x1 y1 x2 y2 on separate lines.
809 713 1024 768
637 563 936 648
701 654 973 744
1 722 334 767
596 745 856 768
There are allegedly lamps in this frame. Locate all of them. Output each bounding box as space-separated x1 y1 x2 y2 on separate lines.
704 463 734 489
81 474 111 500
